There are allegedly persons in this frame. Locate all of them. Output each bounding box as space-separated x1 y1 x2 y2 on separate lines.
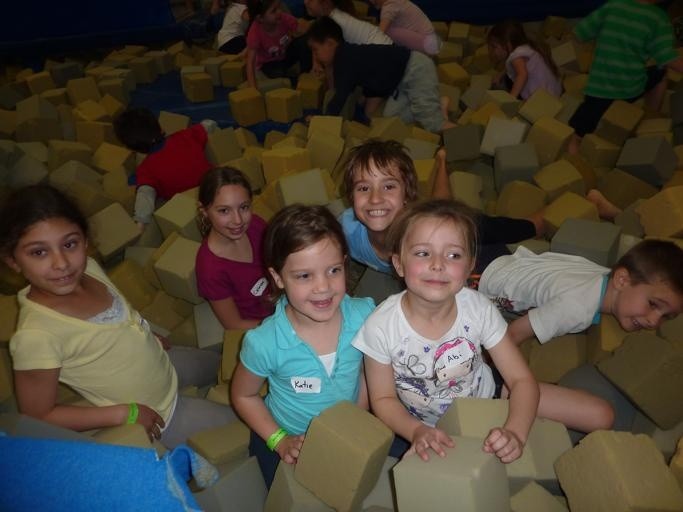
1 184 241 450
231 203 412 489
351 200 615 463
373 1 442 58
246 0 315 89
478 237 683 346
568 0 683 152
487 22 564 101
197 166 271 331
336 140 550 277
114 105 218 230
305 16 458 133
218 0 251 55
304 1 394 44
